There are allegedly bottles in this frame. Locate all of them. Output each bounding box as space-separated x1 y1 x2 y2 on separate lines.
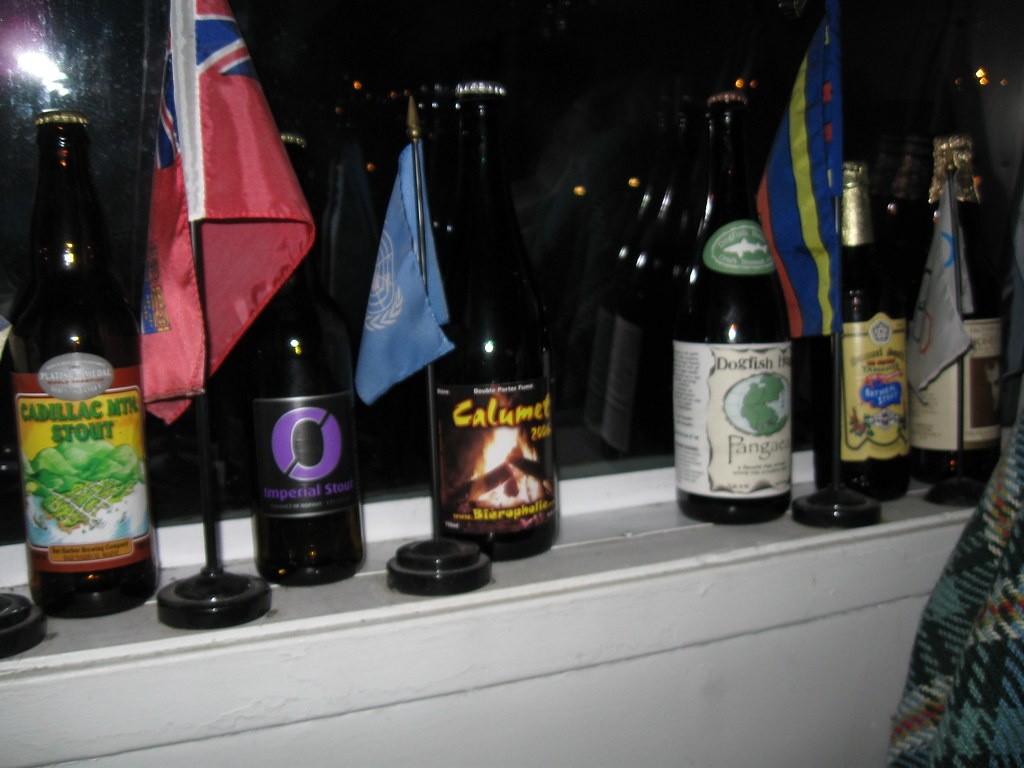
435 71 557 563
908 136 1006 483
7 111 156 618
673 94 793 527
602 191 673 455
816 161 912 502
240 134 364 588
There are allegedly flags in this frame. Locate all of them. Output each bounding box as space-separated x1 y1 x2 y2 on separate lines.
139 0 315 427
757 0 844 340
354 141 456 408
901 170 976 397
